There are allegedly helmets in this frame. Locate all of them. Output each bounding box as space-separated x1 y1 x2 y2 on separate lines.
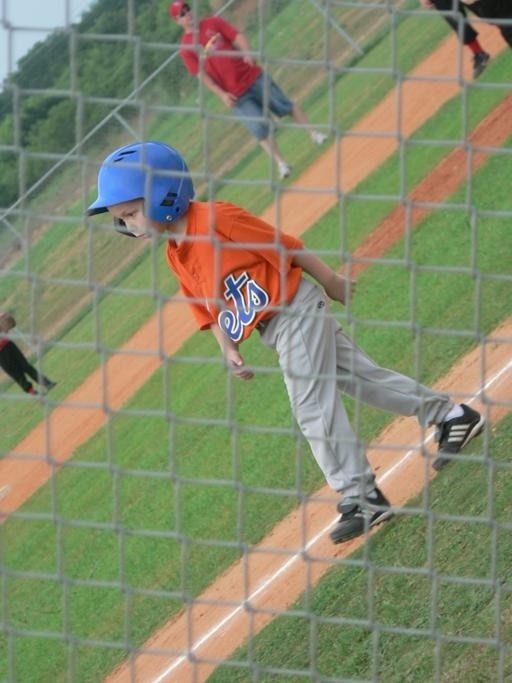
89 141 194 238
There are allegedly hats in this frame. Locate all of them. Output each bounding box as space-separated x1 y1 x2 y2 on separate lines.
169 0 188 15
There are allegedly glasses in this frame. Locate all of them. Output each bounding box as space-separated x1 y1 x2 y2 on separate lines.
181 9 190 15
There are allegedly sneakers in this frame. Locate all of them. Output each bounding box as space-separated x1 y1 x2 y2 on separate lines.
312 130 327 143
331 488 394 544
279 165 290 178
433 404 485 470
472 52 489 77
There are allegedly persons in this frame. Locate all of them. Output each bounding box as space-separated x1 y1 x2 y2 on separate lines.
82 140 485 548
420 0 510 84
169 0 332 178
0 309 58 398
